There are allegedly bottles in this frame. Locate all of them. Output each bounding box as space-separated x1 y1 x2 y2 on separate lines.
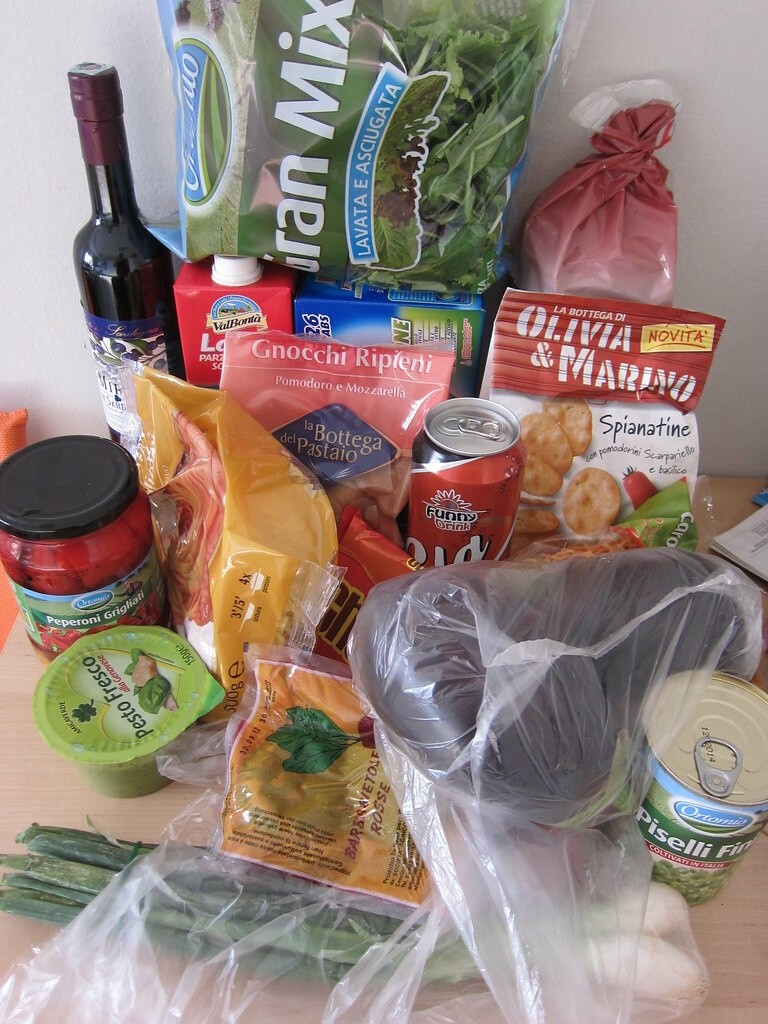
62 57 185 447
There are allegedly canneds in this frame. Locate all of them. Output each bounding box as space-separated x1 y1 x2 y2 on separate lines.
0 434 165 668
405 396 527 568
622 669 767 906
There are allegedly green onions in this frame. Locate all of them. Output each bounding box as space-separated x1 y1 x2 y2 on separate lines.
0 823 705 1004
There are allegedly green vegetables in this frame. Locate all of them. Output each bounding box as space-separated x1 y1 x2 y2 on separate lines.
255 1 563 297
